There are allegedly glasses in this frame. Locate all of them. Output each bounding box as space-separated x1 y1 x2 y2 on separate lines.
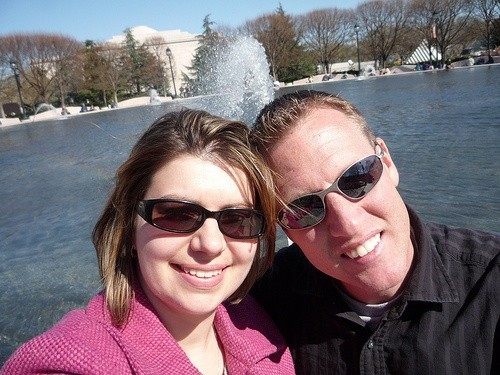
135 198 265 239
277 141 385 231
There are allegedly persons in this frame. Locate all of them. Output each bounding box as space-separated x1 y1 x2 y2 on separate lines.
240 90 500 375
0 107 295 375
